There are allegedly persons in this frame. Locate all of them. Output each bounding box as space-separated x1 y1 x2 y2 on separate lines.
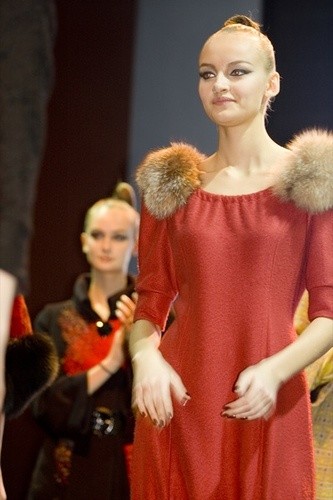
127 14 333 500
290 287 333 500
23 179 142 500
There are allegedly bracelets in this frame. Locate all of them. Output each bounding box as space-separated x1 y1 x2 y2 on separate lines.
98 362 113 376
131 348 161 362
